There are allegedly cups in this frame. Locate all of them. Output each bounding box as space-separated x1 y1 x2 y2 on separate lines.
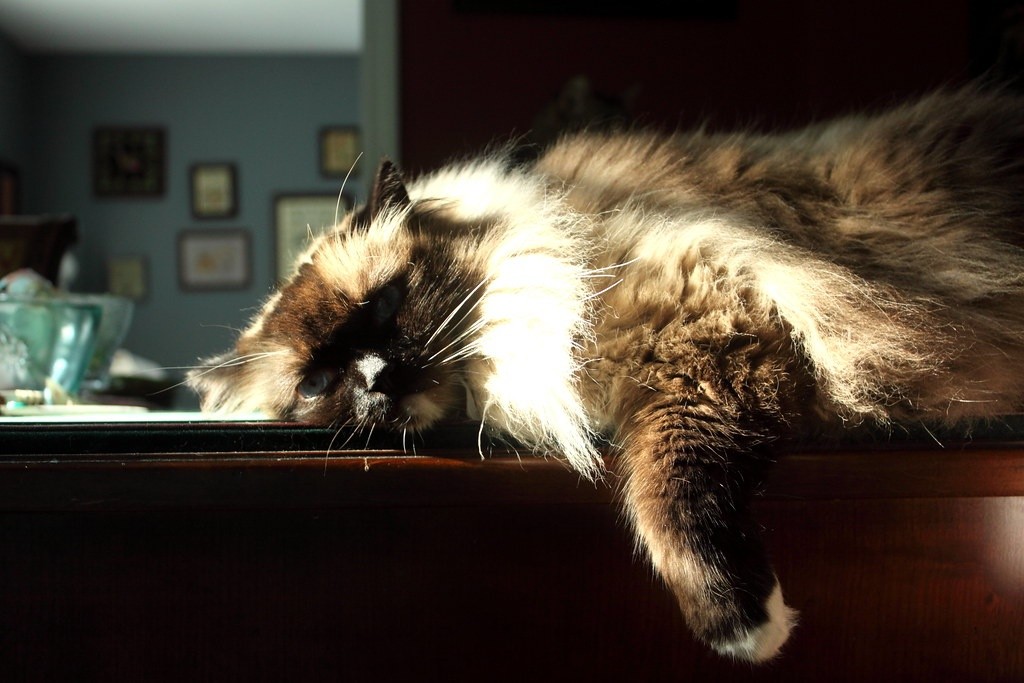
35 294 135 391
0 300 103 405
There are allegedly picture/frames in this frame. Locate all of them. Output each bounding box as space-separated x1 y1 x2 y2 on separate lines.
176 228 252 292
88 123 168 203
188 163 240 221
318 123 359 180
271 190 355 289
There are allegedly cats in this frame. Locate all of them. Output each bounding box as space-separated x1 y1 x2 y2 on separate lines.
182 78 1024 662
